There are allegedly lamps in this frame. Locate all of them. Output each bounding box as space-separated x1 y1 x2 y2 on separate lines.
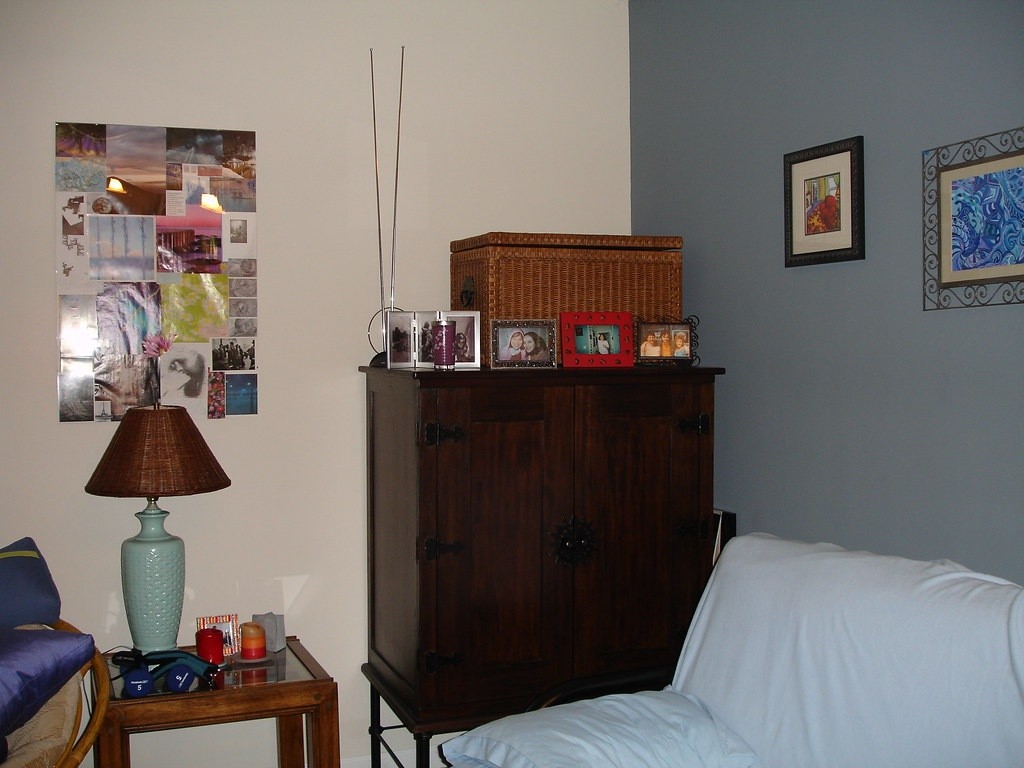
84 404 232 656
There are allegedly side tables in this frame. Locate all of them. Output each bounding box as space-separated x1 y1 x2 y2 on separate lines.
90 635 340 768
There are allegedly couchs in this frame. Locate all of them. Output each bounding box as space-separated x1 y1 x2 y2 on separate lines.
438 531 1024 768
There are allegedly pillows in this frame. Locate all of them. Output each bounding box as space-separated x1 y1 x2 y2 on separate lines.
0 537 62 632
0 629 95 736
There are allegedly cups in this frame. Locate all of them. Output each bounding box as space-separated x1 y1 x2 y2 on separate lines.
196 626 224 665
240 623 266 658
431 320 456 370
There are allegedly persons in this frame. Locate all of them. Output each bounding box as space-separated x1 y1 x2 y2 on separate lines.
231 226 244 241
598 333 610 355
421 321 431 346
500 327 549 360
640 330 686 356
212 338 255 370
213 626 232 650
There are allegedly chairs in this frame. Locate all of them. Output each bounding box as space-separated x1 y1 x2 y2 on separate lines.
0 619 110 768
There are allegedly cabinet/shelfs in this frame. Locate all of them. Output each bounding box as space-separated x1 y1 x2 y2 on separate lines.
358 364 728 768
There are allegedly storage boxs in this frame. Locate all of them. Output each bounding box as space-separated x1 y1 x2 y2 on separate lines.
449 232 683 364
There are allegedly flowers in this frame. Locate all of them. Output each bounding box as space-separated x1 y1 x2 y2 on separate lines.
142 331 176 411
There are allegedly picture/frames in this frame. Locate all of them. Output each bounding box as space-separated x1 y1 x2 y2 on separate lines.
386 311 480 369
195 613 240 657
633 314 701 366
560 312 633 367
922 127 1024 311
783 135 866 267
489 319 557 369
